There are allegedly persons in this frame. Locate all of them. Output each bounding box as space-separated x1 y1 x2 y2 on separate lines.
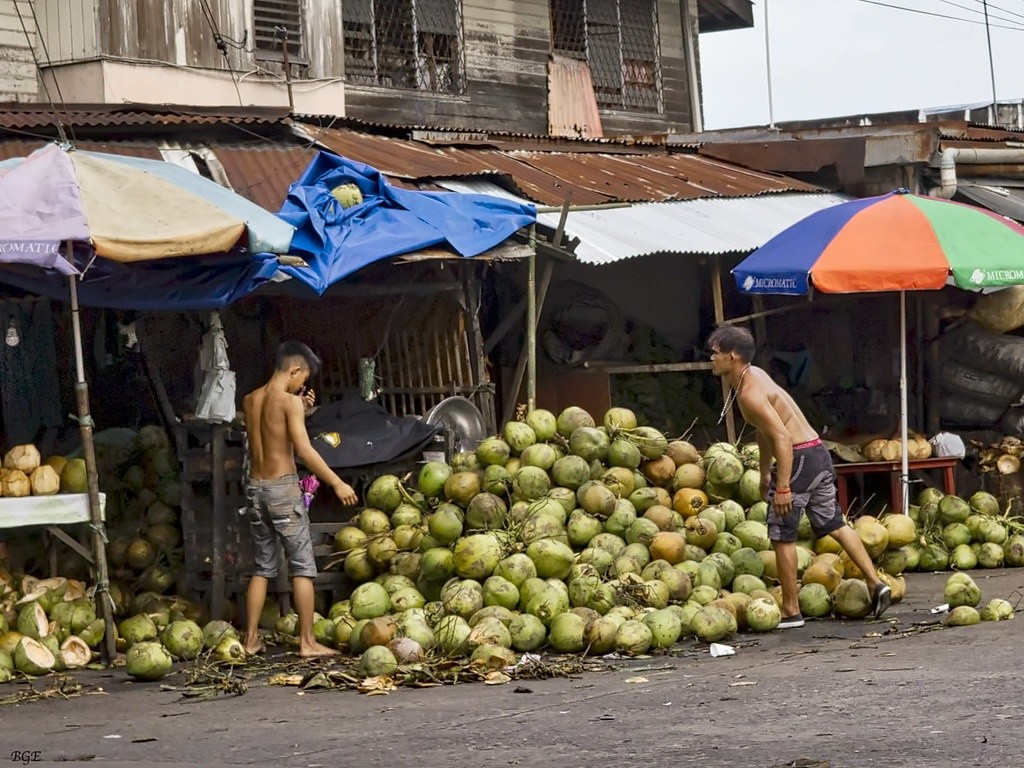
708 324 891 630
242 342 357 656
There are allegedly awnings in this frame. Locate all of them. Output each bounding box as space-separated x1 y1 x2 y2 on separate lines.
0 121 858 265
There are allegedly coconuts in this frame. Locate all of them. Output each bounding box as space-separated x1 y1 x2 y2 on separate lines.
1 407 1024 679
332 184 362 209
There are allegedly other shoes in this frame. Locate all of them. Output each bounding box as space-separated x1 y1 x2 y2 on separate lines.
749 613 805 632
873 584 891 617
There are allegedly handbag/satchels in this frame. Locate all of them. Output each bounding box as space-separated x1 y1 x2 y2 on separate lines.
193 331 239 424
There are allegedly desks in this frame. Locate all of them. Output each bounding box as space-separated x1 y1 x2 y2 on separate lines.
0 492 108 664
833 456 960 517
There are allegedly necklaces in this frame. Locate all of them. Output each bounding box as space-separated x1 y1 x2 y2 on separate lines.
718 364 752 425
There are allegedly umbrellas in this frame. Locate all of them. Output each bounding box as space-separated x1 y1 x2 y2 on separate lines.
729 187 1023 517
0 142 299 668
